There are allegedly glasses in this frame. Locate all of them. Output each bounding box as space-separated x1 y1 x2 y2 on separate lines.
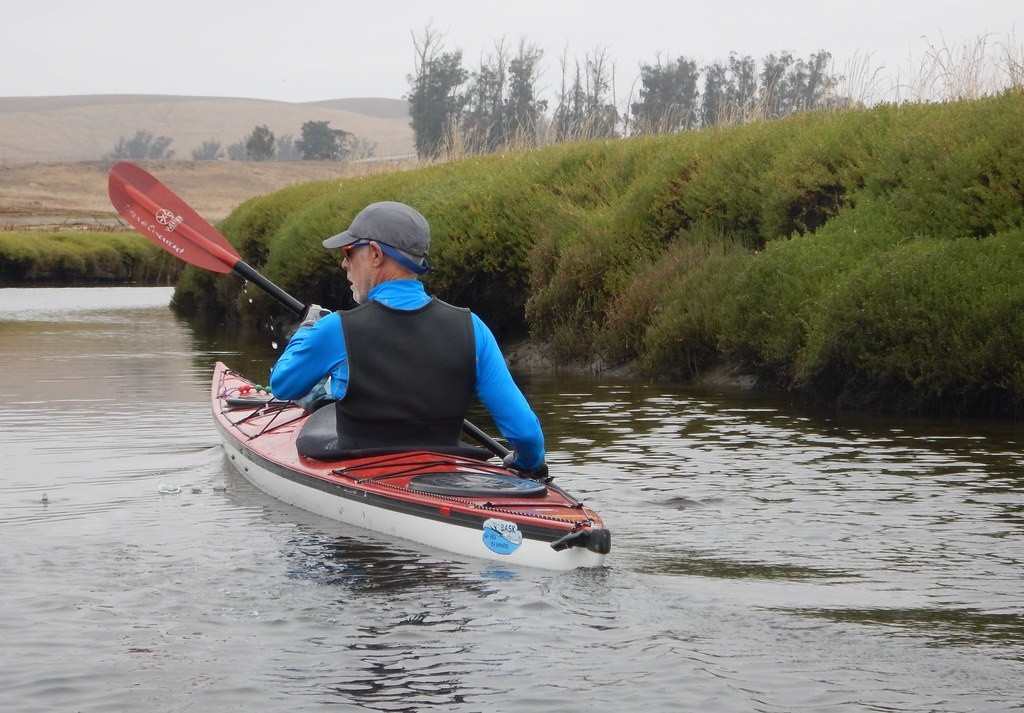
341 241 361 255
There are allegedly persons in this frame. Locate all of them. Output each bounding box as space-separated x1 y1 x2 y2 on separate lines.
268 201 546 479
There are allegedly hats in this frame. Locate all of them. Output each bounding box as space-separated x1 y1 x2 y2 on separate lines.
322 201 431 259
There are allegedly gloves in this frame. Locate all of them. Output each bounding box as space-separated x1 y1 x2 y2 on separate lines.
302 302 333 326
502 450 549 479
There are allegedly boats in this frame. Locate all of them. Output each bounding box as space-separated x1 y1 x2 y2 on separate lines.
210 360 611 571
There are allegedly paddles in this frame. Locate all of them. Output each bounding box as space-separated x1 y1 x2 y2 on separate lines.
107 160 509 462
